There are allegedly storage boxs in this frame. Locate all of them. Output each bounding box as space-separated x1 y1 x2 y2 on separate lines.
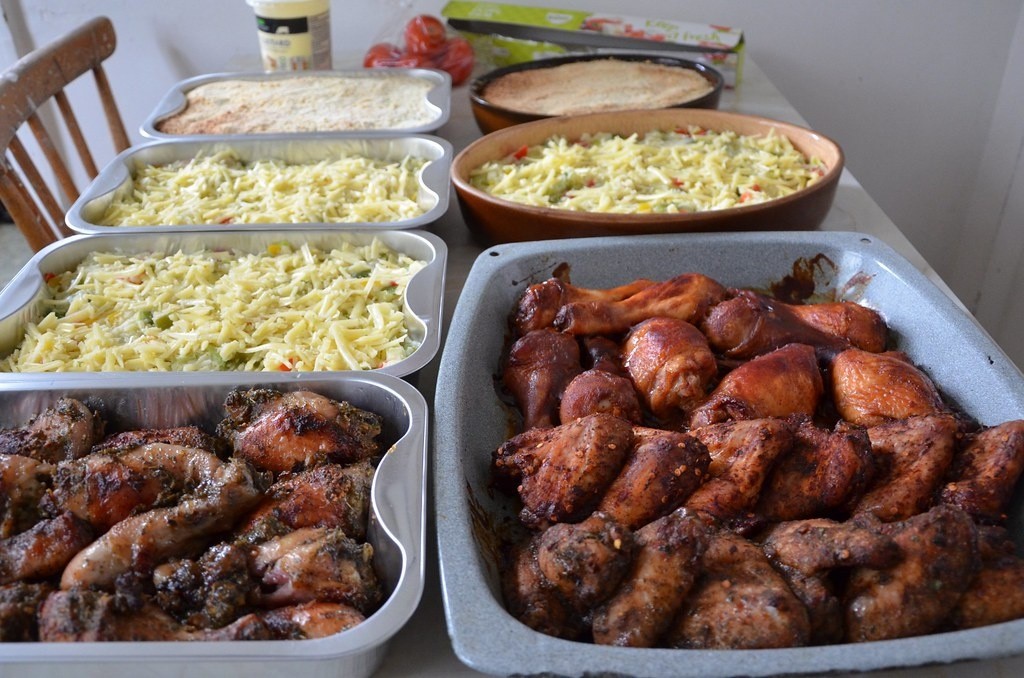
443 1 745 92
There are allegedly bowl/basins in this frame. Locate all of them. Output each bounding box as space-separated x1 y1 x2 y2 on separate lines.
0 41 1022 676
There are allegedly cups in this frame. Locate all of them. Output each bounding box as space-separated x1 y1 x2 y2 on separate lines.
243 1 337 71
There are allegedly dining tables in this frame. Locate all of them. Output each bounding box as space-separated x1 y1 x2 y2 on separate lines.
212 43 1024 677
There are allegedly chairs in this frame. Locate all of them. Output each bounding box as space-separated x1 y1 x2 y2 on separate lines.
0 16 131 259
